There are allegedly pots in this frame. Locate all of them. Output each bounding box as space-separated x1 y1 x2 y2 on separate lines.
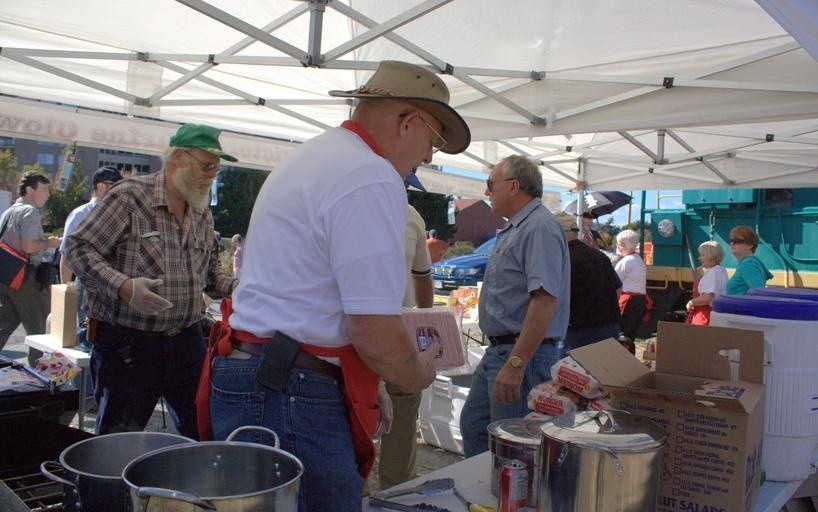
37 425 304 512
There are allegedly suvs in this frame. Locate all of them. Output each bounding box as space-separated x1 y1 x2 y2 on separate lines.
430 235 497 291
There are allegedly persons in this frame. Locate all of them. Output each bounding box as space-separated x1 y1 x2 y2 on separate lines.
425 228 450 264
549 211 623 357
228 231 243 274
361 200 435 495
68 121 239 442
459 151 572 459
576 215 608 252
0 169 63 352
685 239 728 326
723 223 773 299
196 57 472 510
614 230 651 330
58 165 123 329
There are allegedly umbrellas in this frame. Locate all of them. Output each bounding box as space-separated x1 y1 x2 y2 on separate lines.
564 190 634 218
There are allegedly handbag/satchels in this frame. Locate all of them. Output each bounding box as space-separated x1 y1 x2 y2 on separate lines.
0 242 31 291
36 250 75 286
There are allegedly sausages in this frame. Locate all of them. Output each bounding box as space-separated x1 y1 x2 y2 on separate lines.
400 311 465 368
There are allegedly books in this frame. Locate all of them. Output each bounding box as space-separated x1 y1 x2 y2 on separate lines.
400 309 467 370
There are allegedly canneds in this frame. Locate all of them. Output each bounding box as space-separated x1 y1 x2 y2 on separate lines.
500 459 529 512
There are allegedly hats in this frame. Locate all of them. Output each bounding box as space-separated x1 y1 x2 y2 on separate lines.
326 58 471 156
169 122 238 163
92 165 122 183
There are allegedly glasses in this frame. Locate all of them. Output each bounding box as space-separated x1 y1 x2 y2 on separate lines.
184 149 224 176
400 109 448 153
486 178 514 191
729 240 745 245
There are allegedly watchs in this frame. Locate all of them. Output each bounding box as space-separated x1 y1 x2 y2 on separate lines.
505 353 527 369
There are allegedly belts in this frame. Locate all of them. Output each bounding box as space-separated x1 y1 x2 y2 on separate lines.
488 335 556 344
235 335 343 376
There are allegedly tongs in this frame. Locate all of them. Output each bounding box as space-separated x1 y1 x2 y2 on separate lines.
368 476 456 512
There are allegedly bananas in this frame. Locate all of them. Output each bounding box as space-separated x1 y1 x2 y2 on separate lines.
468 504 496 512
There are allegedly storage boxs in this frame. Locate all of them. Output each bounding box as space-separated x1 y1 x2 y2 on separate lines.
567 321 767 511
